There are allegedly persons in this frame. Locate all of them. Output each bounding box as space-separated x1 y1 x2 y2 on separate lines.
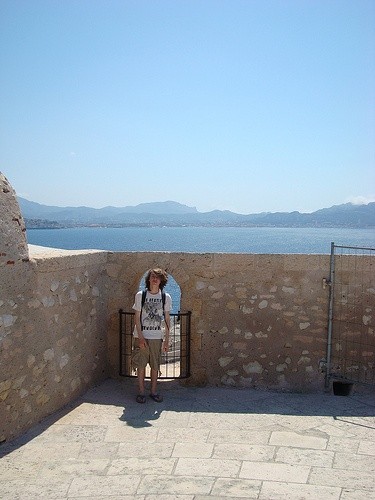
133 266 171 404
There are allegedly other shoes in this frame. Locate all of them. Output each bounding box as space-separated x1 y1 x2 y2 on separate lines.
136 394 146 403
150 392 163 403
